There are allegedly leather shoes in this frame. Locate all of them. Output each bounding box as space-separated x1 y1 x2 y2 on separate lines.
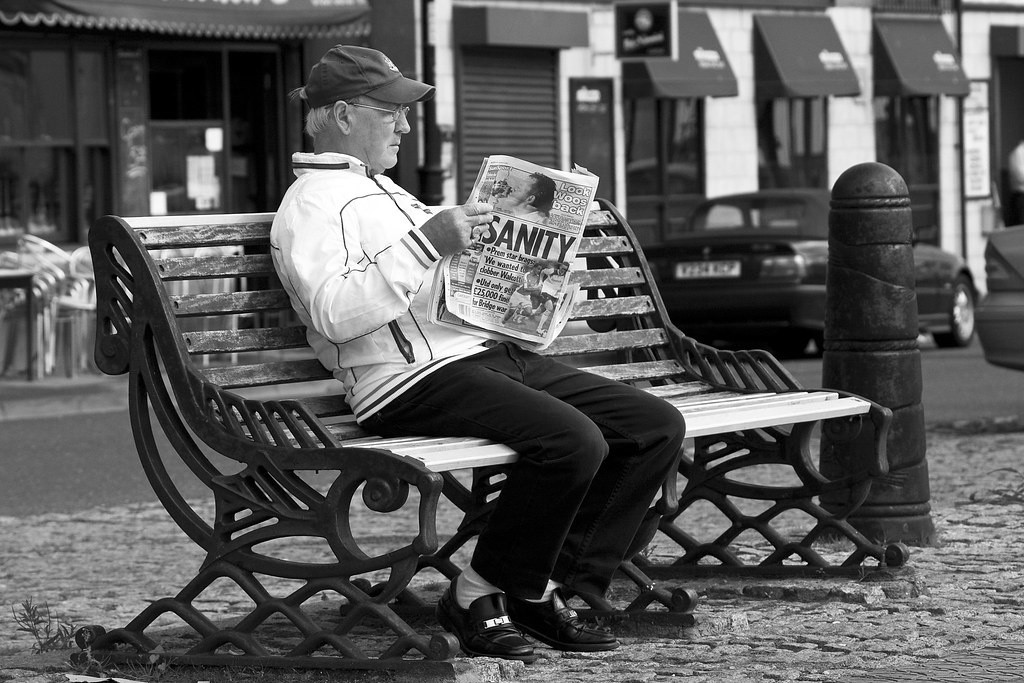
435 574 538 662
506 587 620 652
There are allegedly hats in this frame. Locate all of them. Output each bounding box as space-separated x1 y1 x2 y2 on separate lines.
301 44 436 111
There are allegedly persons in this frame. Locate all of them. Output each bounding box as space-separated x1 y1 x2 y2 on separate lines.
506 172 557 224
273 46 684 659
502 261 570 336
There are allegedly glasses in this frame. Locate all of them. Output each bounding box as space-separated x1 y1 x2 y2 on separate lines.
323 102 410 121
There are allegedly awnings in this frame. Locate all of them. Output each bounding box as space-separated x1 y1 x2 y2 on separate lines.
873 16 970 98
0 0 374 38
754 14 860 98
622 11 737 98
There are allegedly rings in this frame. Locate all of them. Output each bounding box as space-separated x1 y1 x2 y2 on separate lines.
471 226 481 238
472 239 475 245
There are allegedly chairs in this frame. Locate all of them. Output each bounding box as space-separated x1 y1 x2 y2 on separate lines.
0 228 250 377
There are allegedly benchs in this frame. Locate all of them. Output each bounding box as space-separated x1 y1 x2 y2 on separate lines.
69 198 914 665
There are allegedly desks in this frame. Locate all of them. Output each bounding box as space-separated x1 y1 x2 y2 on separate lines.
0 267 37 383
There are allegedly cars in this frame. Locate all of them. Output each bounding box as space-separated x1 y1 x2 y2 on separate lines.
653 187 980 356
